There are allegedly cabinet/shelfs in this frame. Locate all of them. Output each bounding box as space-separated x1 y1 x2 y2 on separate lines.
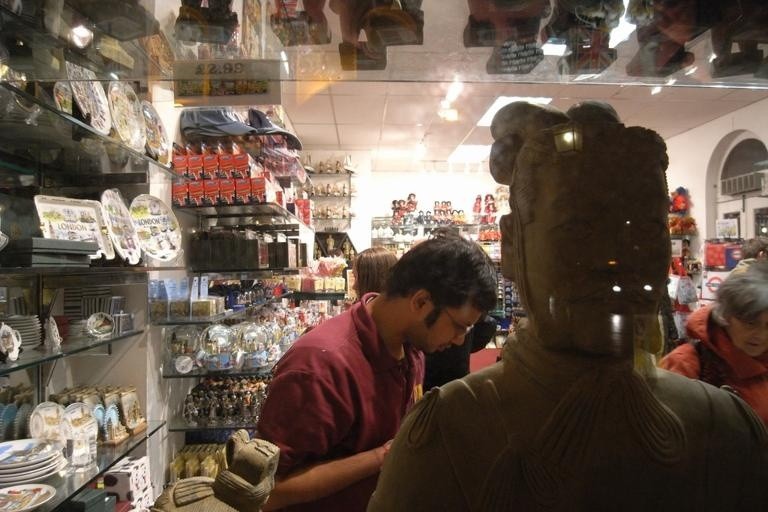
0 3 355 511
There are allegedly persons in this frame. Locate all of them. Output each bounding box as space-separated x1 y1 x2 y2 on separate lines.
423 227 495 395
152 428 279 512
740 234 768 258
661 258 767 423
353 246 398 304
369 104 768 512
254 234 496 511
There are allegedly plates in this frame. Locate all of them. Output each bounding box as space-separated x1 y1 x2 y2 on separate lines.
1 401 102 510
33 189 184 269
66 52 172 163
87 312 116 341
0 314 62 361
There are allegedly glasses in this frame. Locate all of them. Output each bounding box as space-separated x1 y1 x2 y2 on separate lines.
443 308 474 336
